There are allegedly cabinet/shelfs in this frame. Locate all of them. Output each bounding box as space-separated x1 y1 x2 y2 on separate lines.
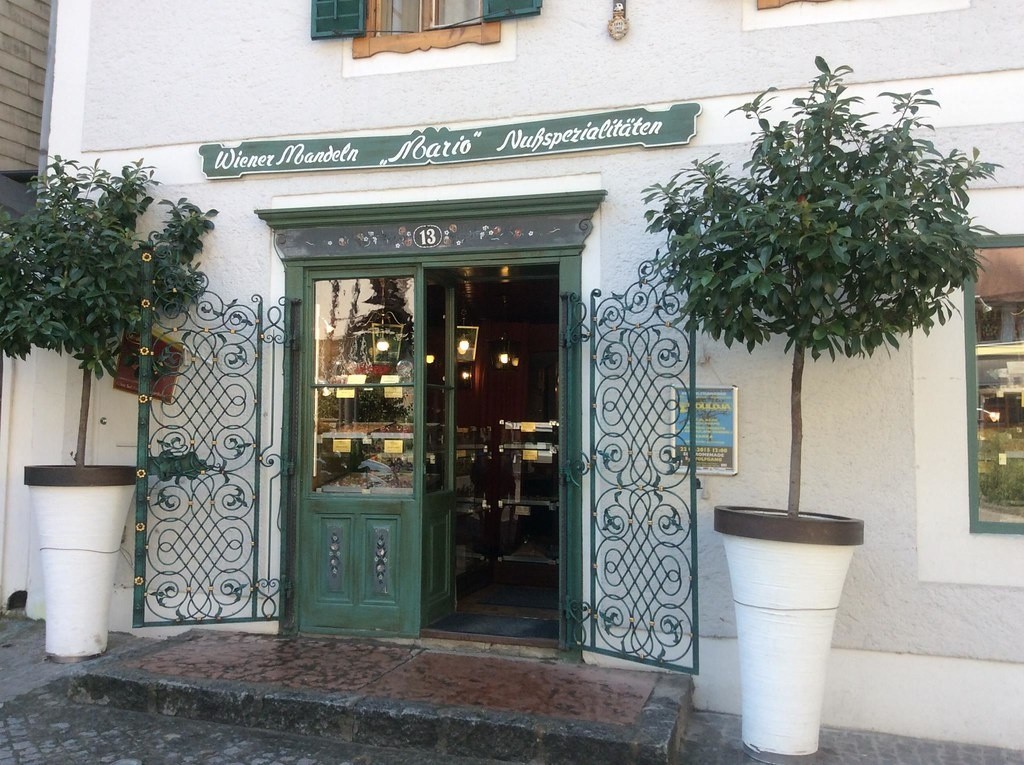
312 381 558 613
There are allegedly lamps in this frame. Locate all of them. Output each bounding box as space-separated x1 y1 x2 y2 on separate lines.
371 265 523 370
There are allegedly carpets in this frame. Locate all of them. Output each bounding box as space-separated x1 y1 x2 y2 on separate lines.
461 583 560 610
428 611 559 641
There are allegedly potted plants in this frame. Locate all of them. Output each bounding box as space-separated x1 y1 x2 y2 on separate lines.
639 54 1003 757
0 157 214 664
